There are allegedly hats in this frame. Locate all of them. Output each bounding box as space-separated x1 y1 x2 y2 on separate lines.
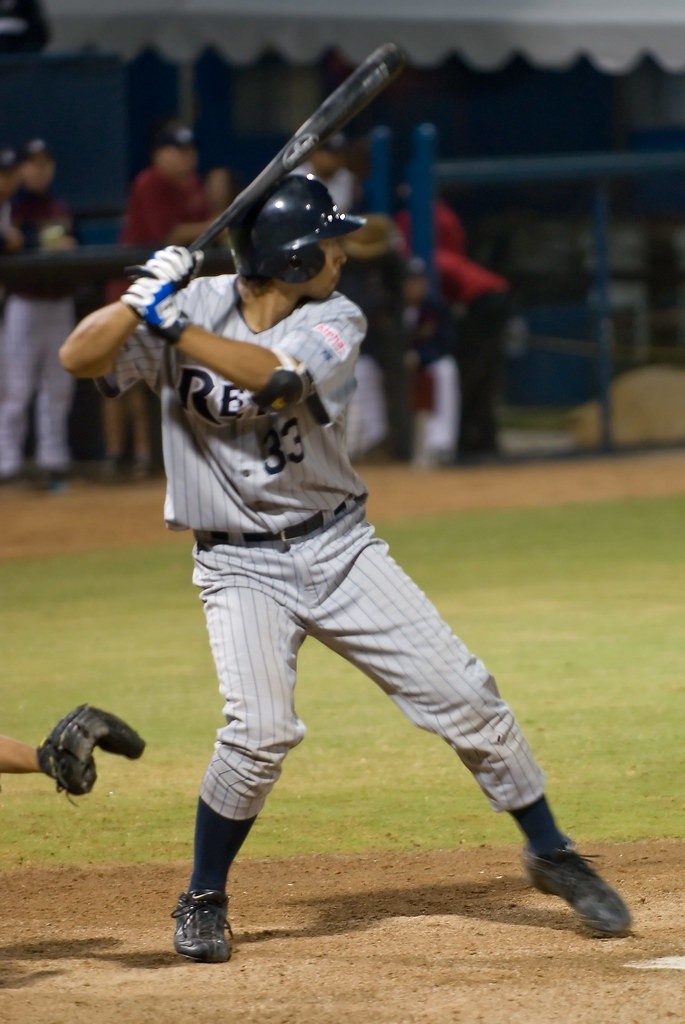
166 130 196 149
1 148 18 173
28 139 53 156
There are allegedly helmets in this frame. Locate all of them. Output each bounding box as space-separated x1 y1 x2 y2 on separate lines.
227 172 368 283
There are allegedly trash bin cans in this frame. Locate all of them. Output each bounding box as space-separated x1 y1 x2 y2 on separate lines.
507 302 595 408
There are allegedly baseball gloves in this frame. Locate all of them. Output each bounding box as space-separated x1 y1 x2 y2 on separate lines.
35 701 146 809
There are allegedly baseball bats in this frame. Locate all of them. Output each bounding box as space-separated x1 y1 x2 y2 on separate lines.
126 37 412 322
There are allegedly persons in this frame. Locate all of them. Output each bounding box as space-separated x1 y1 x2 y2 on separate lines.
0 126 520 495
58 175 632 965
0 704 147 799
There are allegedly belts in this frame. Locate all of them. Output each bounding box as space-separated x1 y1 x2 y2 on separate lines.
193 500 346 542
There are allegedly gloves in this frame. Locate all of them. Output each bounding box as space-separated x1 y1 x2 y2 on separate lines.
121 278 189 344
136 244 205 290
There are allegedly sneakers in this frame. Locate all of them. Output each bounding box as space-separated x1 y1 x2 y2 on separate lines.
521 840 632 936
170 889 234 962
